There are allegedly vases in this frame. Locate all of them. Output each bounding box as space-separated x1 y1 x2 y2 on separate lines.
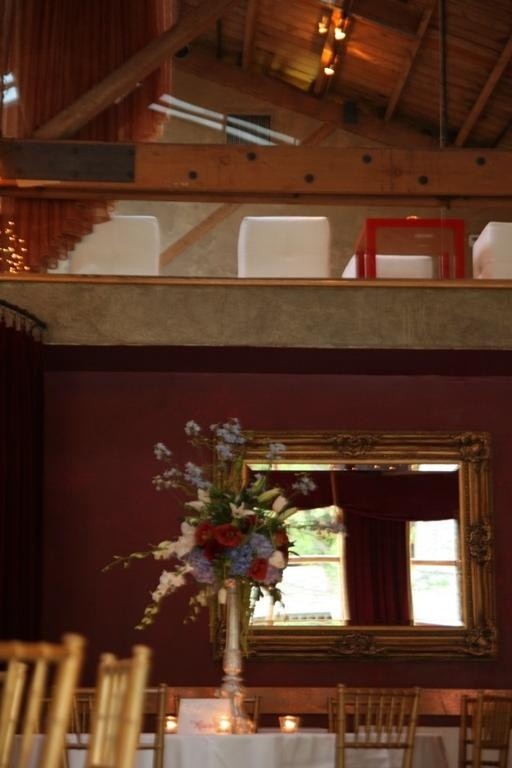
213 576 258 732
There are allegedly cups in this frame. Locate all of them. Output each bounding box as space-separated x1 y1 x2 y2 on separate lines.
279 714 301 734
212 714 235 735
162 715 178 733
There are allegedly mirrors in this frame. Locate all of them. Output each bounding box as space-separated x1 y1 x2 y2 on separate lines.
208 430 502 660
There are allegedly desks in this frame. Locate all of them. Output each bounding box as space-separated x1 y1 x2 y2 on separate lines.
354 218 466 279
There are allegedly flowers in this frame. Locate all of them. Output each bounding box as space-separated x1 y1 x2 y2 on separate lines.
102 418 340 658
